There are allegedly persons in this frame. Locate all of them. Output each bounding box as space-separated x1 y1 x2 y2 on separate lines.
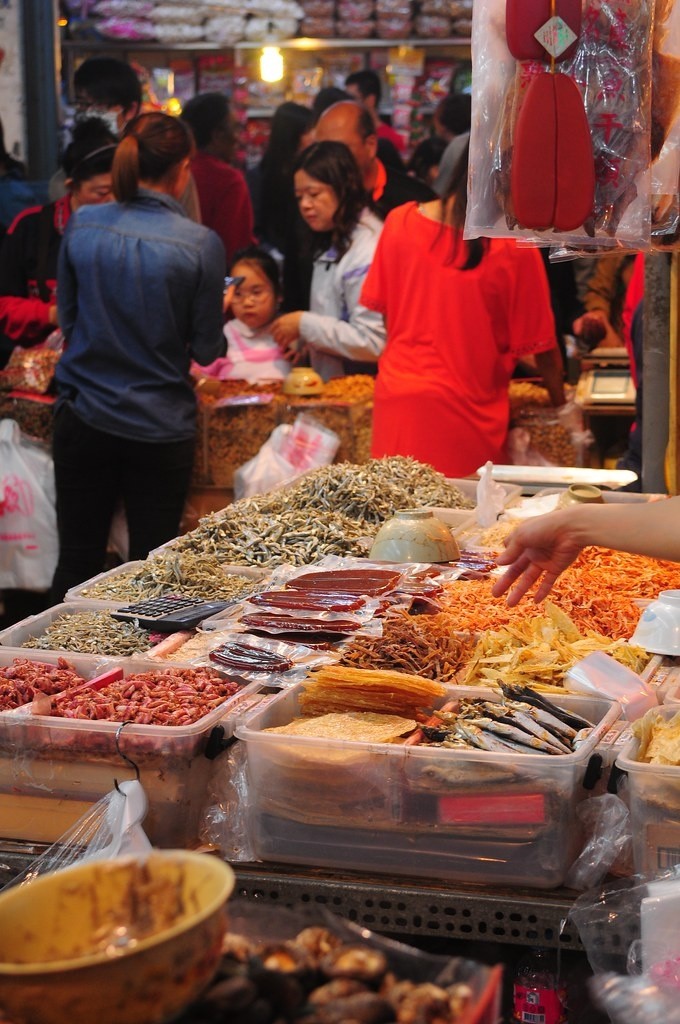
492 492 680 611
1 58 642 608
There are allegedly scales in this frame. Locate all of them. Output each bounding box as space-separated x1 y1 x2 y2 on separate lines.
573 347 639 406
475 461 639 500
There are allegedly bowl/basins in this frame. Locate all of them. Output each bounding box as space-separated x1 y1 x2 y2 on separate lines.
558 483 606 509
0 851 235 1024
282 368 325 395
369 508 461 562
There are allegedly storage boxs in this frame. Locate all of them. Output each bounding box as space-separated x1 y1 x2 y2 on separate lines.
0 477 680 889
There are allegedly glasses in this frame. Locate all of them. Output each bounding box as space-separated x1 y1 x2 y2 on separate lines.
74 100 118 114
230 287 274 304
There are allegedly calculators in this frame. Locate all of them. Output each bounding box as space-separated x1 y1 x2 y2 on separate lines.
111 593 236 635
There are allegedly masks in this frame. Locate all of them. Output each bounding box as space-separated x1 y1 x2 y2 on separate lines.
86 109 127 135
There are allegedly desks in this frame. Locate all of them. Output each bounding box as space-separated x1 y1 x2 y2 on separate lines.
574 369 636 469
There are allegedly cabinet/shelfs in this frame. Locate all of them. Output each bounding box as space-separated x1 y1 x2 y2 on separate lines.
64 37 471 169
